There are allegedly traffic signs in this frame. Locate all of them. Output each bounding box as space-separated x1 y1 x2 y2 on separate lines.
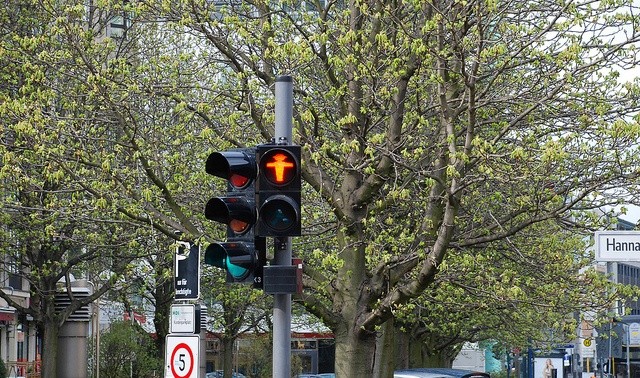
584 339 591 346
172 231 200 301
165 335 200 378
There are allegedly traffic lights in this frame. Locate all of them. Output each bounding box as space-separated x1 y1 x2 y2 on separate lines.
257 144 302 237
204 147 266 287
